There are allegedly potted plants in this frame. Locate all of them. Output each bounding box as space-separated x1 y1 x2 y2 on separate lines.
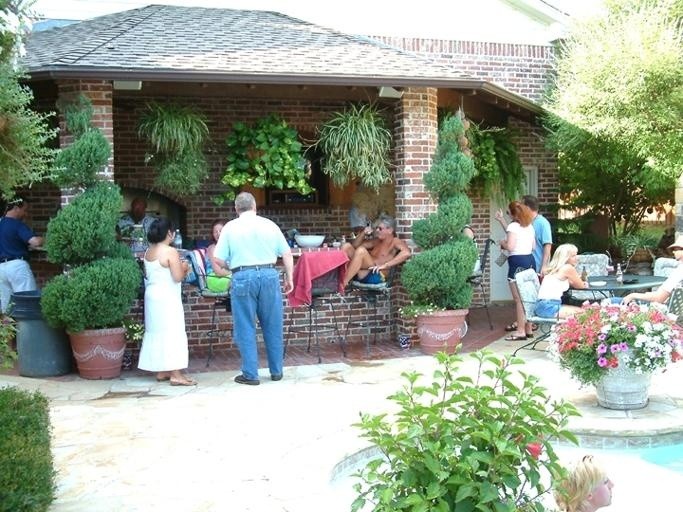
211 112 316 207
38 93 145 381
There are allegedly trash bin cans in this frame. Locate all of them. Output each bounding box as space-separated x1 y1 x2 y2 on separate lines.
7 290 72 378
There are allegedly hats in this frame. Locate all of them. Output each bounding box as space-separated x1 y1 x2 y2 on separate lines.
667 236 683 256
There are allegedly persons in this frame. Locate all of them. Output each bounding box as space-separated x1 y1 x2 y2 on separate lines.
536 244 590 320
136 214 199 386
599 236 683 311
213 191 295 386
459 223 480 284
119 198 158 257
494 200 535 341
203 219 232 295
0 197 46 356
339 216 411 292
344 180 371 237
552 450 614 512
495 194 552 332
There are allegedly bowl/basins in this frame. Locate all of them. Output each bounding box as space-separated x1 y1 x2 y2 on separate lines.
293 235 326 247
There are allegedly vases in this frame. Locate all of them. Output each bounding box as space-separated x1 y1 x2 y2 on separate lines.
595 348 651 411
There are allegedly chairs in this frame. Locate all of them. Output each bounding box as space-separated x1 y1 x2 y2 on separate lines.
465 238 496 331
184 248 395 367
511 253 683 356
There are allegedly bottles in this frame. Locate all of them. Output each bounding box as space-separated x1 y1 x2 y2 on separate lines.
173 229 182 249
580 266 587 281
614 263 623 286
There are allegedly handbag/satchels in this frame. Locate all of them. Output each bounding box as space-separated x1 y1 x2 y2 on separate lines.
495 253 508 267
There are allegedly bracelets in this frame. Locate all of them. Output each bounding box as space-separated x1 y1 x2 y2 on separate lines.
384 262 391 269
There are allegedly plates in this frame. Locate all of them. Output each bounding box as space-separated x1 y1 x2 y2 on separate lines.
589 281 607 287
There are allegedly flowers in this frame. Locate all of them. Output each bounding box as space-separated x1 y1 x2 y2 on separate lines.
118 318 145 341
398 113 478 356
398 300 447 318
545 299 683 390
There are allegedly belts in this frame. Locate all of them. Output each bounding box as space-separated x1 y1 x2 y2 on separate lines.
232 264 275 273
0 256 29 263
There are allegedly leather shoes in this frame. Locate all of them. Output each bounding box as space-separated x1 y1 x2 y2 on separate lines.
170 375 198 385
235 376 259 385
157 373 170 381
272 375 282 380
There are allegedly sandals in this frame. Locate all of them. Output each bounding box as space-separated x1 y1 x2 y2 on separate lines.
505 321 538 340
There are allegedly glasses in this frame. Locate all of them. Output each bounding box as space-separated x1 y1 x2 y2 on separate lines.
583 455 593 463
376 227 384 231
